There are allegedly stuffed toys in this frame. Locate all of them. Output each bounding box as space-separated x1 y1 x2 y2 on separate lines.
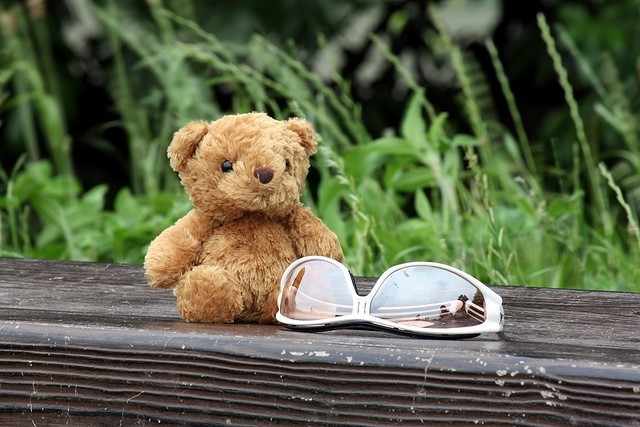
143 110 346 324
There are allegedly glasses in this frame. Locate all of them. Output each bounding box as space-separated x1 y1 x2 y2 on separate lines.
274 255 503 340
